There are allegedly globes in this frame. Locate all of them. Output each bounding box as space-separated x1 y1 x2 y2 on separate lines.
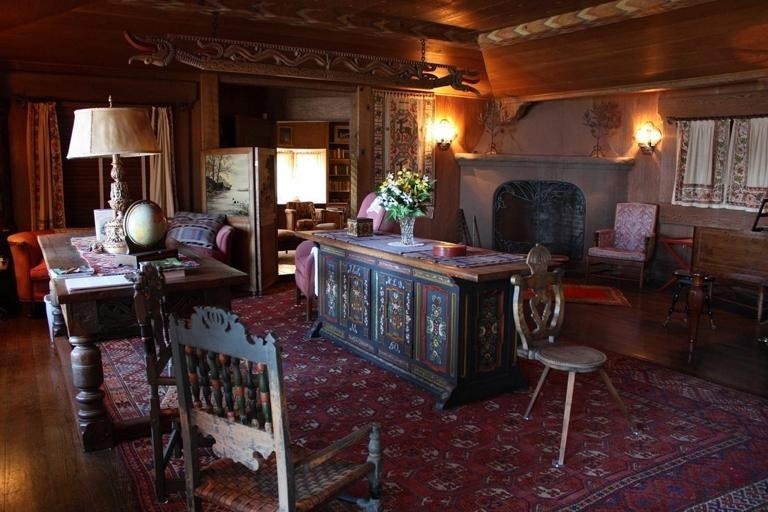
115 199 178 270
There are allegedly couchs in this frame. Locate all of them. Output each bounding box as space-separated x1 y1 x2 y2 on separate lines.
7 226 93 318
274 201 348 253
165 217 233 264
292 192 396 323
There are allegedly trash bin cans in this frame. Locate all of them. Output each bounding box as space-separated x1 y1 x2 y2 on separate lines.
44 294 55 343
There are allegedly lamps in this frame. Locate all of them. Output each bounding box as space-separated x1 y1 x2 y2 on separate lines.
433 119 457 151
66 96 162 249
631 121 662 155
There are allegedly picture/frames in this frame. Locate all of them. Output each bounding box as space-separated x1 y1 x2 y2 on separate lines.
275 125 294 147
333 124 350 144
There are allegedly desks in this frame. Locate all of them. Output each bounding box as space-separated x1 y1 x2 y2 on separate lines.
293 229 570 410
656 233 693 288
35 229 249 454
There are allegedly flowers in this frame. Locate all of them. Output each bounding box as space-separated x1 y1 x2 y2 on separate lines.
376 168 437 225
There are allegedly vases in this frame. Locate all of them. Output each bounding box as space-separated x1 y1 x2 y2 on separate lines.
398 216 417 246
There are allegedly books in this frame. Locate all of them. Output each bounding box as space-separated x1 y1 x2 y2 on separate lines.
48 258 199 295
329 148 350 204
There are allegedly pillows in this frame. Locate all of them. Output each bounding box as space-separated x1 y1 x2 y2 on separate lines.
167 210 225 249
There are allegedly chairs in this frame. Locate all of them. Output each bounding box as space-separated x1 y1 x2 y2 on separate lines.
584 201 658 289
162 305 385 511
509 243 640 465
123 261 264 504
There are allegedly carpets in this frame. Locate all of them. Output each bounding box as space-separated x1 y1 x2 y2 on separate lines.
94 282 766 512
551 282 632 307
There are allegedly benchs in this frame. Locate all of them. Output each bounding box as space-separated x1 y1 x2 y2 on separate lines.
689 226 766 325
